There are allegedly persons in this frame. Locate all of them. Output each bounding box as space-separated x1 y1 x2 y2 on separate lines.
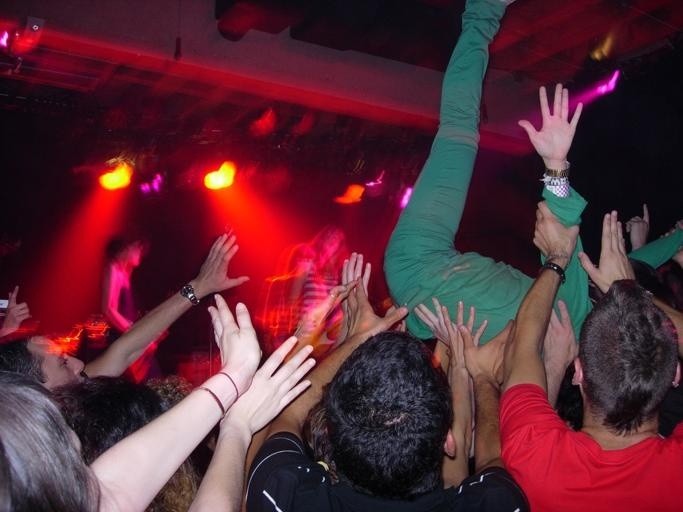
0 1 682 510
